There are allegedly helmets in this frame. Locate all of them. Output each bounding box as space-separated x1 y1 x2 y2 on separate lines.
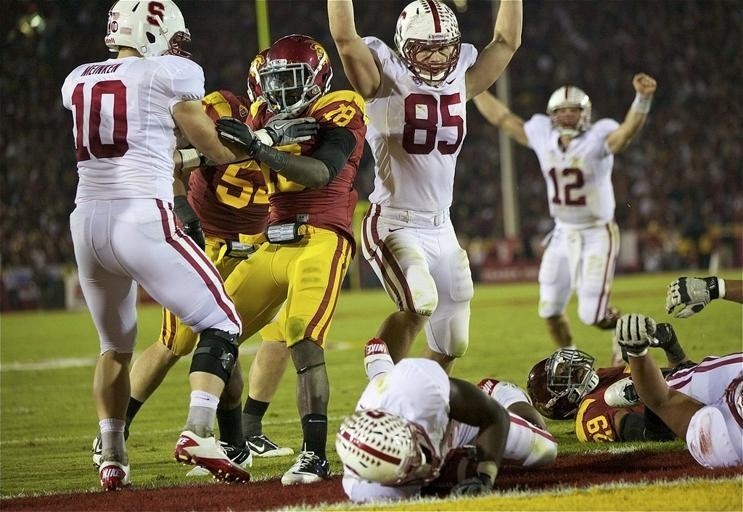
105 0 193 59
394 0 462 85
527 349 600 421
248 35 334 119
335 408 437 485
547 86 592 139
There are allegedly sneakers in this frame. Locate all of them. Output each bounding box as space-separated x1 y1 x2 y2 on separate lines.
174 430 253 483
478 379 533 407
363 337 394 376
604 376 643 408
91 431 130 491
247 434 294 458
280 451 331 486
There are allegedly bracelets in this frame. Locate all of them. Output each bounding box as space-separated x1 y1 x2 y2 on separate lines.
478 463 498 482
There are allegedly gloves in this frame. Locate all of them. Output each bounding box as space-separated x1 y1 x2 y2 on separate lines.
650 323 677 351
666 276 719 318
617 313 656 357
451 478 492 496
215 116 262 158
265 113 320 146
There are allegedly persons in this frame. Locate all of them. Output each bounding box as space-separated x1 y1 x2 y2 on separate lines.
334 337 556 505
528 276 743 472
471 73 657 371
59 0 318 491
215 34 367 486
92 47 294 459
0 0 743 275
326 0 524 381
